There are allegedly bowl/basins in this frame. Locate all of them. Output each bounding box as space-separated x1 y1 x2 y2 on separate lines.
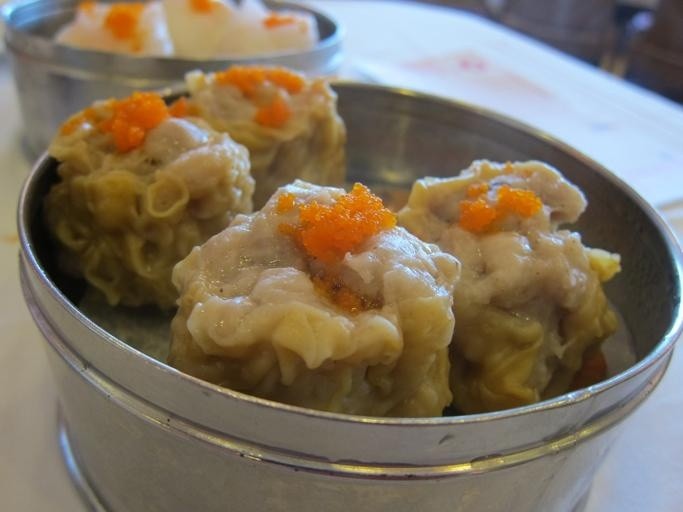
0 0 345 167
11 73 682 512
